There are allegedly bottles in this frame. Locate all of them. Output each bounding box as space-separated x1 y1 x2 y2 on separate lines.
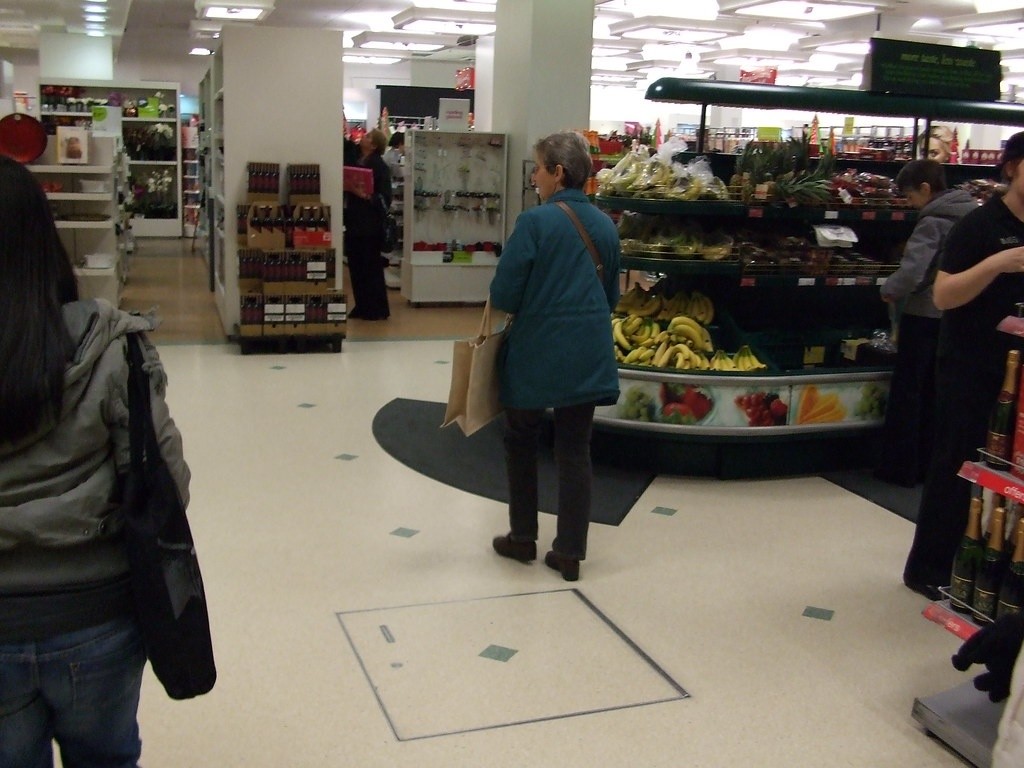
948 349 1024 627
237 161 347 325
468 112 474 124
583 176 598 202
574 128 601 155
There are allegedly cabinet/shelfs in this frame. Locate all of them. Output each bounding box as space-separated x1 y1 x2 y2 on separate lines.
25 135 132 308
234 189 347 353
921 316 1024 643
399 130 508 308
182 160 200 238
343 163 404 287
198 25 343 344
37 78 182 237
545 77 1024 480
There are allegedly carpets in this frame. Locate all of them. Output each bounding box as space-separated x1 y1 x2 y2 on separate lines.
371 397 659 526
823 467 925 522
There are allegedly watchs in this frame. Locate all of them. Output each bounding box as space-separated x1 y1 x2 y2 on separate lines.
364 193 368 200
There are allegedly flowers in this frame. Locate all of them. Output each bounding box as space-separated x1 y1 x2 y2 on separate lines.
41 85 174 119
131 169 174 205
122 120 175 161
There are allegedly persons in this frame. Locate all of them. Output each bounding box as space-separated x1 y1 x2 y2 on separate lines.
878 131 1024 602
915 126 957 163
952 609 1024 768
343 128 405 320
0 154 190 768
493 131 621 579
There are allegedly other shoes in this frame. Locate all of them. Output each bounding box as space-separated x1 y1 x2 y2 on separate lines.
493 532 537 562
903 574 951 601
545 551 579 581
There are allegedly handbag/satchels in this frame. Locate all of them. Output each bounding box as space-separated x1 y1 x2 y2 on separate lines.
440 294 510 437
114 334 216 701
380 216 394 253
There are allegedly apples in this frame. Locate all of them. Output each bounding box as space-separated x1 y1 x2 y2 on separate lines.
659 383 712 419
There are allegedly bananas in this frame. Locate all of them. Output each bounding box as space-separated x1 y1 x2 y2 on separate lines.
611 281 769 372
597 155 735 260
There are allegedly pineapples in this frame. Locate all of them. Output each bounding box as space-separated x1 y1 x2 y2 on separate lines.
725 133 833 202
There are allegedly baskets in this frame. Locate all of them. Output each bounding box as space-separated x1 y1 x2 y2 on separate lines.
142 132 176 161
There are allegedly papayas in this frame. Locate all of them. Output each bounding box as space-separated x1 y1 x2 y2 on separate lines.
794 387 848 424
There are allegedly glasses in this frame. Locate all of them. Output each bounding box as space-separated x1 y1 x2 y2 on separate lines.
535 164 569 173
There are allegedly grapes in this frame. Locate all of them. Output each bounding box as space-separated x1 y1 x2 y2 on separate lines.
614 386 694 425
852 383 890 421
735 391 776 428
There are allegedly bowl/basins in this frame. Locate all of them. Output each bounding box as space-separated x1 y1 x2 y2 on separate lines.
85 253 112 268
81 180 105 191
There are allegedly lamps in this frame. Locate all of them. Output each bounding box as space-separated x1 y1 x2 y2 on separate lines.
190 1 1024 89
